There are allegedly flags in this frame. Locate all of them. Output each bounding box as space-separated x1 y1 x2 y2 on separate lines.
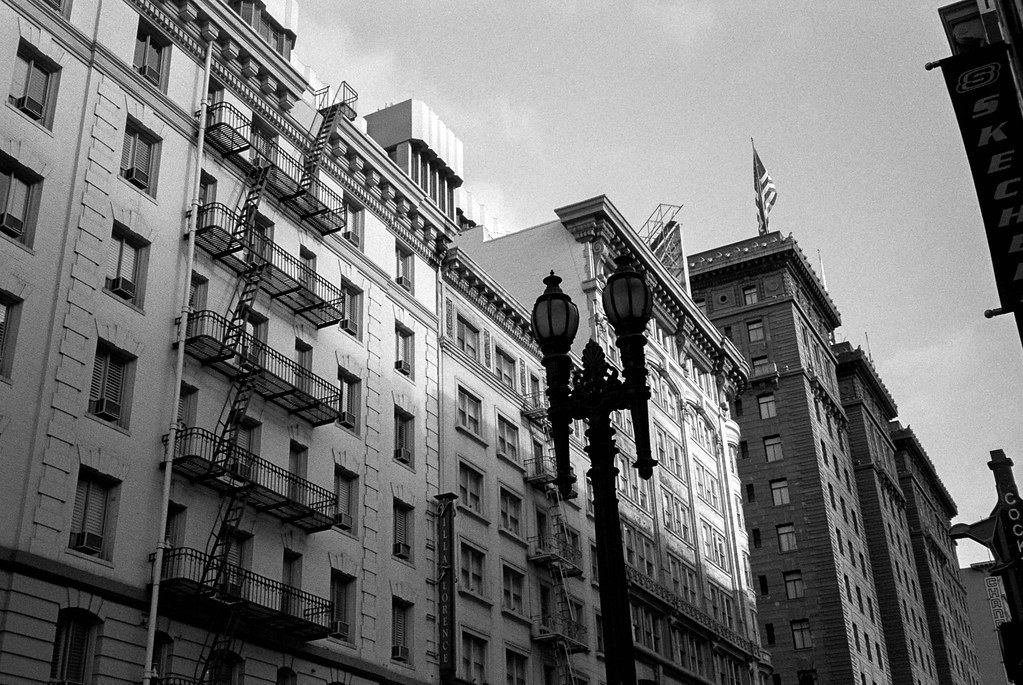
753 148 777 237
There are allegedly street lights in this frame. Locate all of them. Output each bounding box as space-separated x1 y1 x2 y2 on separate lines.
530 248 659 685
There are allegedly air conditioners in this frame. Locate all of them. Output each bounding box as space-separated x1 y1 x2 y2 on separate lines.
247 252 266 267
338 411 356 429
329 620 350 638
74 530 105 554
111 275 137 300
392 542 410 559
339 318 358 336
394 447 411 463
240 352 261 372
137 64 162 86
343 230 360 248
124 166 149 190
0 211 25 239
334 512 354 530
391 645 410 661
396 275 411 291
231 461 253 480
16 94 44 121
251 155 271 169
395 359 411 376
94 396 123 422
218 580 242 598
